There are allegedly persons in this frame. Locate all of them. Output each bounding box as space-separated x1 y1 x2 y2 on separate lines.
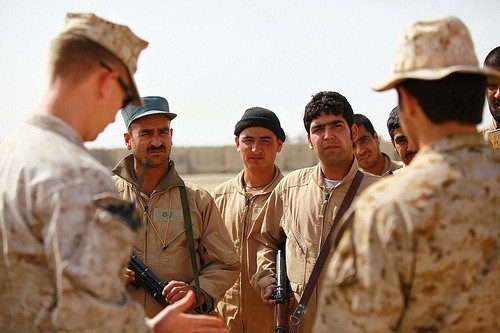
0 12 497 332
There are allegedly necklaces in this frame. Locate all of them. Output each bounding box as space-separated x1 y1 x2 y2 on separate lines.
134 187 173 248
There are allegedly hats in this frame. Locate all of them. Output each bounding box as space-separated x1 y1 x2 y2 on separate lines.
371 15 499 91
60 11 150 106
234 107 286 142
120 95 177 130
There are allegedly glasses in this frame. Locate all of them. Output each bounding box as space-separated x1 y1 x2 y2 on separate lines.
100 61 133 108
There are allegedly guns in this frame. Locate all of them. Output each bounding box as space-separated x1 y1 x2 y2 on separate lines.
274 250 289 333
128 253 177 307
